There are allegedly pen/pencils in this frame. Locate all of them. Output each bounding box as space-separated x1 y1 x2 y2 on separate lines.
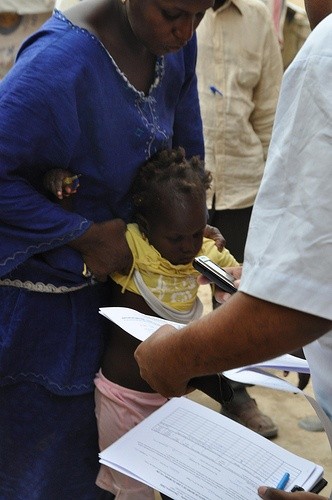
276 473 290 491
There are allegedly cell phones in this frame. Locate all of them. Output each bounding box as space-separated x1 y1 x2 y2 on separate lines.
192 255 239 292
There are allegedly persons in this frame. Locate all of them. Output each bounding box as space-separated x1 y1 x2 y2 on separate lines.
258 484 331 500
0 0 227 500
38 143 242 500
129 1 331 433
190 0 313 440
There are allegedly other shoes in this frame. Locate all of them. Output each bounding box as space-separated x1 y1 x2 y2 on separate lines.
297 414 326 433
217 405 278 439
